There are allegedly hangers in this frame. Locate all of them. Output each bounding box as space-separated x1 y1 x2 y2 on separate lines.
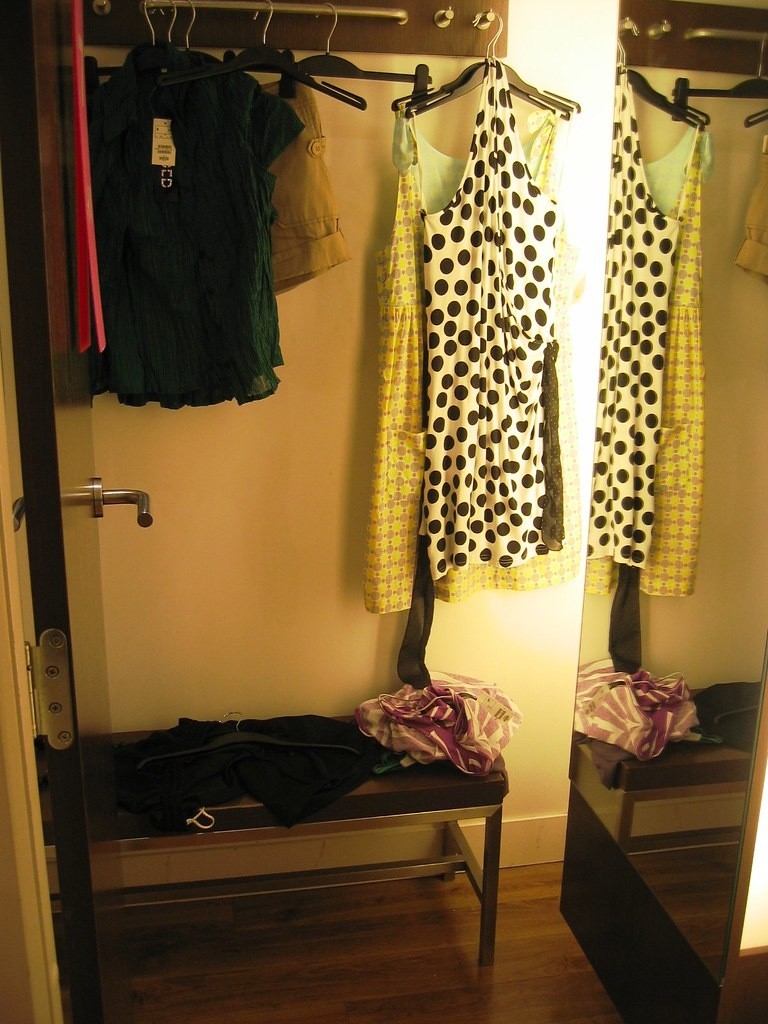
125 1 578 119
609 15 768 131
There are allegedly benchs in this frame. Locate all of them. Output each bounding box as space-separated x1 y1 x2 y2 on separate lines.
33 712 511 967
588 689 756 862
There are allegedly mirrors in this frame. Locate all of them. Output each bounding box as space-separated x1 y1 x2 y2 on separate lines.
609 2 766 690
